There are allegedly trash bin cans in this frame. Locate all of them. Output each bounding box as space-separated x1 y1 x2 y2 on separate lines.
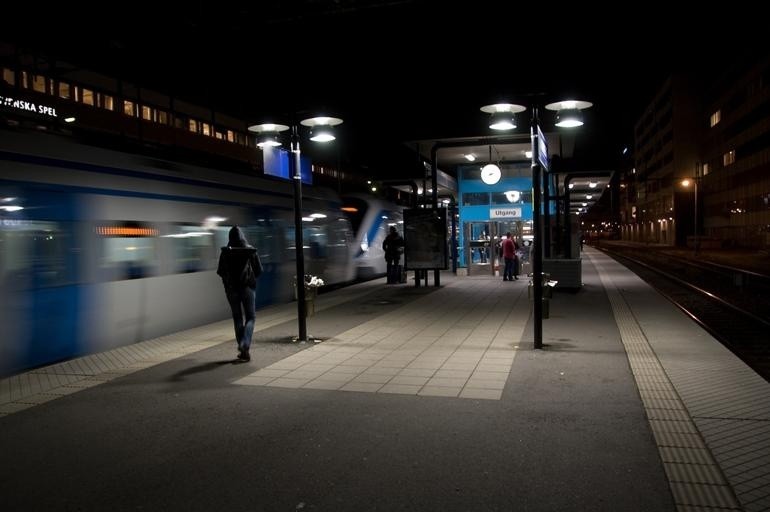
527 272 553 319
293 274 319 317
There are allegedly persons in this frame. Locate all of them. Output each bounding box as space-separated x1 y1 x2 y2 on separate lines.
382 225 404 284
448 230 519 281
580 230 584 251
216 224 265 363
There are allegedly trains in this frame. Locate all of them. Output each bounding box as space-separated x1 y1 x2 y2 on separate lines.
0 126 406 381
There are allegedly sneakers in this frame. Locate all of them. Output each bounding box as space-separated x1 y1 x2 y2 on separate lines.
237 346 250 360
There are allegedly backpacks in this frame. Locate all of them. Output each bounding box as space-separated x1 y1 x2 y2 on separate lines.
220 245 257 288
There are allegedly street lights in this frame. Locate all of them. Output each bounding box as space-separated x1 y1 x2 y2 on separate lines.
620 184 629 225
479 95 597 350
247 109 344 344
683 178 699 256
